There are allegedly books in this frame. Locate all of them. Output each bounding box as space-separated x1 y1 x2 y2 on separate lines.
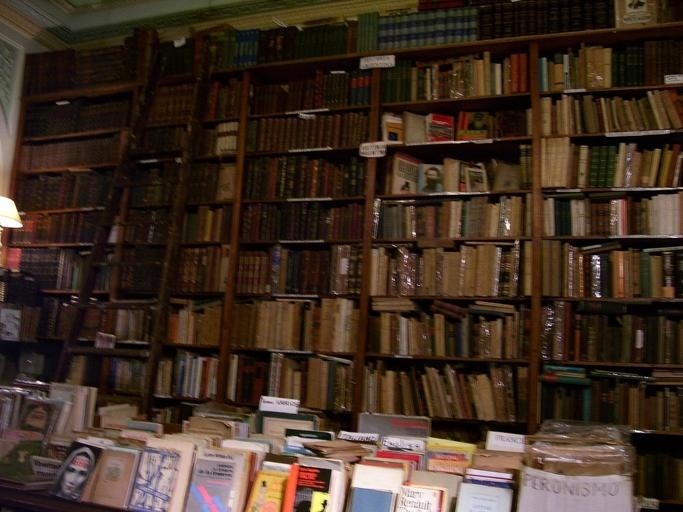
0 0 681 511
